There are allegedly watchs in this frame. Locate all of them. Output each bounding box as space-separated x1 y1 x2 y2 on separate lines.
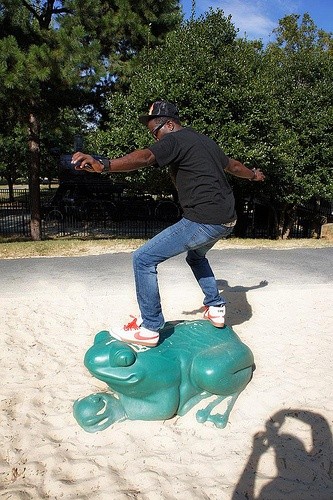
99 159 110 172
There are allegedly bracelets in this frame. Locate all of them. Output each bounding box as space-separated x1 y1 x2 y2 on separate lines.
249 169 255 180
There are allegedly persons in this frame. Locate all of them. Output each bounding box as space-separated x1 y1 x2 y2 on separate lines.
70 101 265 347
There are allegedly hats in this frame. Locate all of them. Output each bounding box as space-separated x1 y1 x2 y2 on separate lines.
139 101 179 125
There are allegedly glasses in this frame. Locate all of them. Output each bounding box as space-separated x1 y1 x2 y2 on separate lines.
152 119 168 139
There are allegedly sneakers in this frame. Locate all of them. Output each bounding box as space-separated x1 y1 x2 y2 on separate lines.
203 305 226 328
109 317 159 347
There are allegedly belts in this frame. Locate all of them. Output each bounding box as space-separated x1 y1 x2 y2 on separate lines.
221 220 237 227
